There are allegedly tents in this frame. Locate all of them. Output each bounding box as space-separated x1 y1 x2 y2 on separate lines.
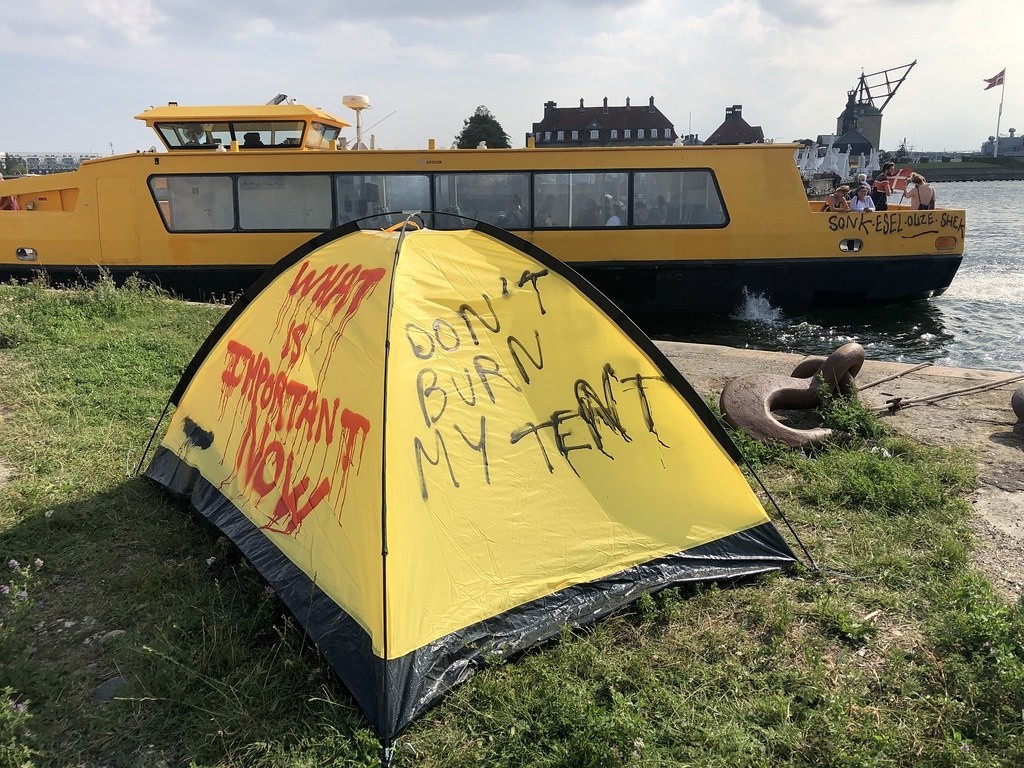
144 209 798 746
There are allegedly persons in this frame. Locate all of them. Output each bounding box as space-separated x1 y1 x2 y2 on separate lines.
903 172 936 210
508 195 523 222
575 194 622 226
0 173 20 211
636 195 667 225
851 187 875 213
871 162 894 211
537 196 555 227
821 186 851 212
858 174 871 193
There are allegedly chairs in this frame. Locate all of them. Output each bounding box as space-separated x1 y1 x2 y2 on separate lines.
244 132 263 146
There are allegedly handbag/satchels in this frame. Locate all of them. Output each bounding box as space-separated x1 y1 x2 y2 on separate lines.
918 204 929 209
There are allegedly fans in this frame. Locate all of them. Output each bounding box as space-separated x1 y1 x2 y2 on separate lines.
183 123 204 144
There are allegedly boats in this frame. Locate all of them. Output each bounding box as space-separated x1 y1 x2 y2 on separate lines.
0 59 970 332
